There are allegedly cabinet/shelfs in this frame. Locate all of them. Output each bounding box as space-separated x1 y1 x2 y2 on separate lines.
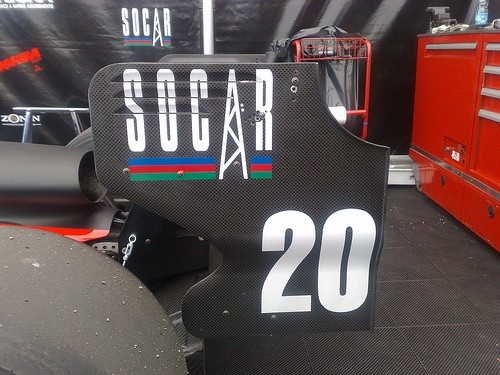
409 30 499 254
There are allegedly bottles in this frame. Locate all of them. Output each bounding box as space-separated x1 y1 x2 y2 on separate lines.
474 0 489 27
